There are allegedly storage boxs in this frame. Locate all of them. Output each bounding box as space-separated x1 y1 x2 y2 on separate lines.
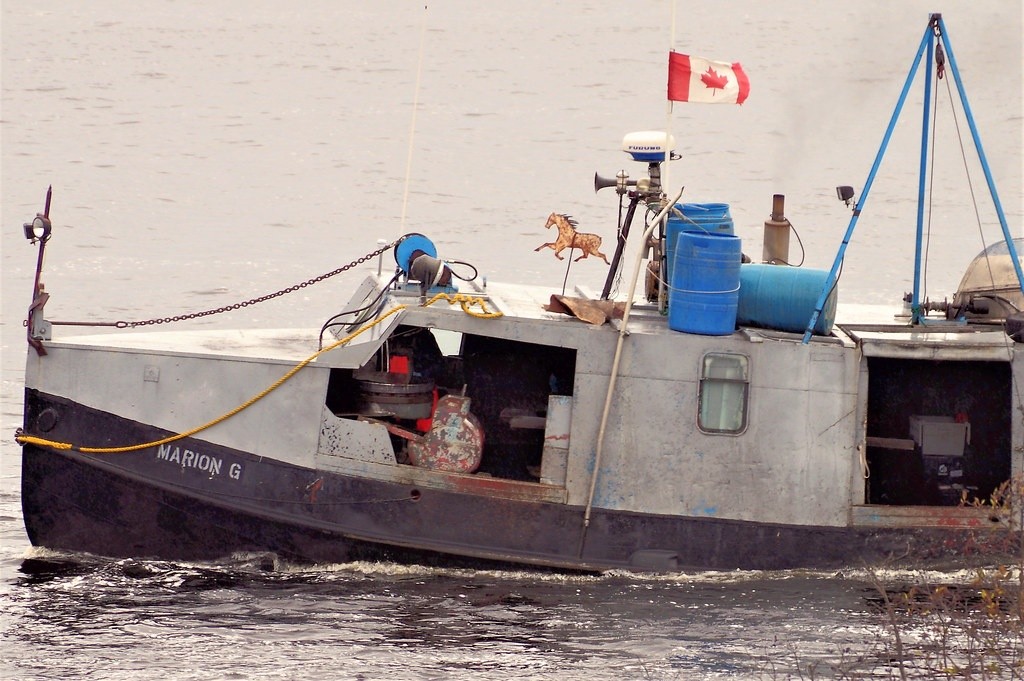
909 413 972 457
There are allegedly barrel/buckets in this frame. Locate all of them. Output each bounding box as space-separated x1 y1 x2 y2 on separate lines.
358 379 435 419
667 217 734 301
670 203 729 218
739 263 837 335
667 230 742 334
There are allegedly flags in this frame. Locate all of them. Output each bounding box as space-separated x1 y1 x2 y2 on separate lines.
668 51 750 104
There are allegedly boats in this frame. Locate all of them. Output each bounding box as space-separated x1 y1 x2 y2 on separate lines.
12 13 1024 575
